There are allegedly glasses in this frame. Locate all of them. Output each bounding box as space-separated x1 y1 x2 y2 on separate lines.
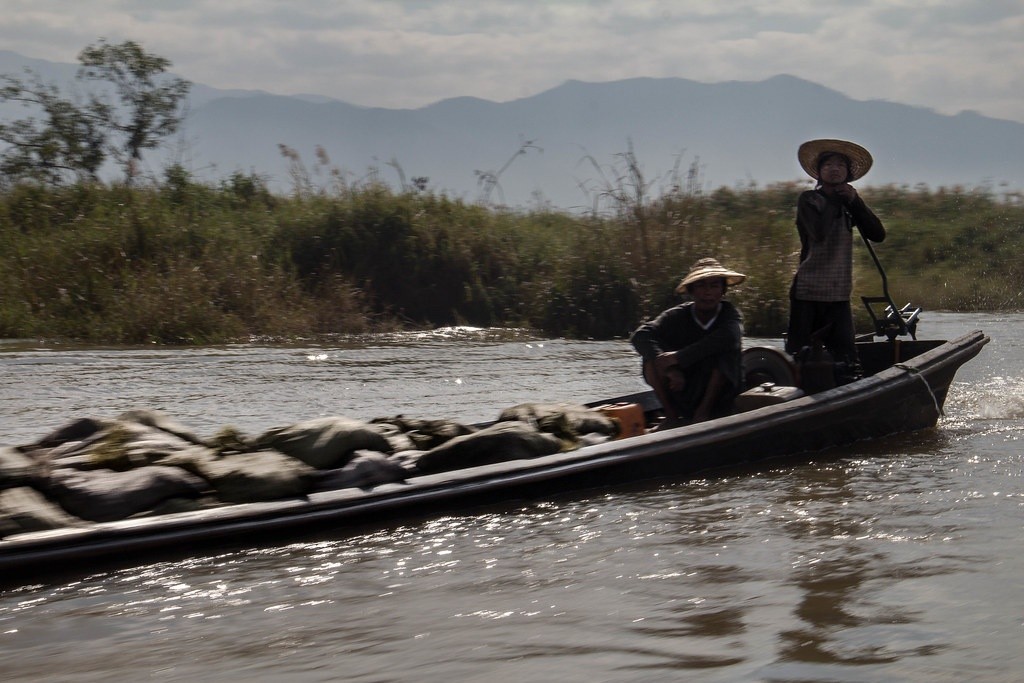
819 160 848 170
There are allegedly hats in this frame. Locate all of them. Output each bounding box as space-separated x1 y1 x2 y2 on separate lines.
676 258 747 293
799 141 872 181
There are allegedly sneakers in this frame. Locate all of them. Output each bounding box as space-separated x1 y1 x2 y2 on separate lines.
651 407 682 431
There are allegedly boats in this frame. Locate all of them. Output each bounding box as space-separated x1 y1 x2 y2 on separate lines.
0 328 992 589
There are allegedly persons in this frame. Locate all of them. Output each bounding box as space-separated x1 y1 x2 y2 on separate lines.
784 139 885 392
629 258 747 431
